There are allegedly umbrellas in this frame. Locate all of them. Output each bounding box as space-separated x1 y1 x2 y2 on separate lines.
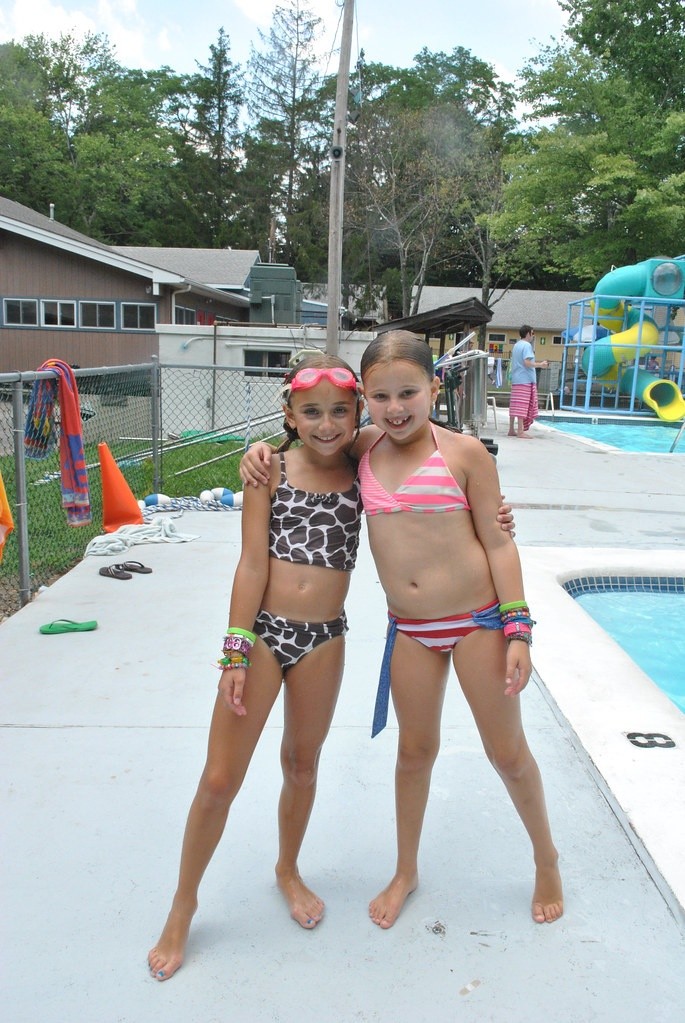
561 321 619 380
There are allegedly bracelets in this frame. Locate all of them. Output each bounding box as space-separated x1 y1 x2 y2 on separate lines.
498 600 534 645
217 627 256 670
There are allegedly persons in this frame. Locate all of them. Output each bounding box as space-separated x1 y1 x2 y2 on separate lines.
146 353 517 980
507 325 549 438
238 328 565 931
648 356 659 370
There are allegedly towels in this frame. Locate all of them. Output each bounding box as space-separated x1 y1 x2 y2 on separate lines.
84 516 203 560
496 358 502 388
488 357 494 375
0 471 14 564
23 357 93 526
509 382 538 431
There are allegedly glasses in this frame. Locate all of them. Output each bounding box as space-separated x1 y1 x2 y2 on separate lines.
292 367 356 393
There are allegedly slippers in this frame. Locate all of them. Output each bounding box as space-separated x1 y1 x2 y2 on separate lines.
39 619 97 634
99 564 132 580
116 560 152 574
